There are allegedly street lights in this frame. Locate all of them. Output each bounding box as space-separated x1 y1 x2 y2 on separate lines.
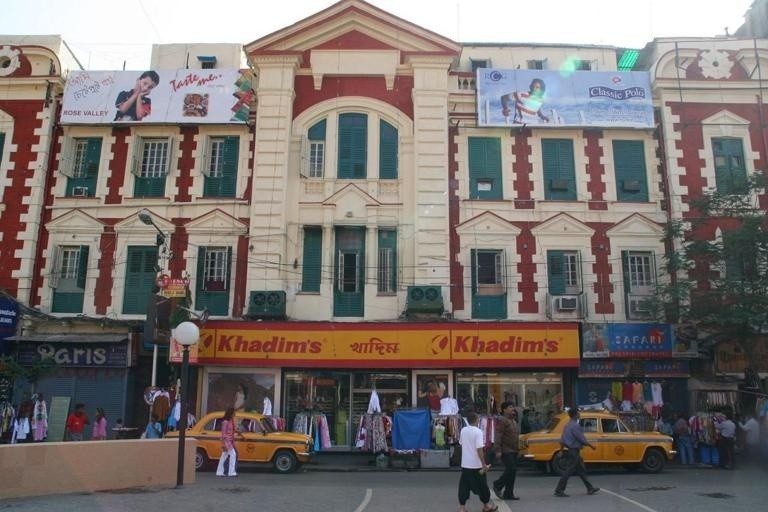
136 212 171 426
172 320 199 491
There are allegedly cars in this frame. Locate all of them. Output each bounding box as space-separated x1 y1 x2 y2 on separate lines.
516 412 676 475
166 410 315 474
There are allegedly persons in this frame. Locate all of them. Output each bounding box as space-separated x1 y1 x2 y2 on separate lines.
501 80 550 125
494 401 520 500
521 406 766 495
145 414 162 438
92 408 107 440
114 71 159 122
232 383 247 412
66 404 90 441
417 377 444 421
458 412 498 512
115 418 126 428
216 407 244 477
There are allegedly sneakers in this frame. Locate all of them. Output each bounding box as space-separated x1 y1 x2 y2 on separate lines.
588 488 600 494
483 505 498 512
553 490 569 497
503 497 520 500
494 481 502 499
